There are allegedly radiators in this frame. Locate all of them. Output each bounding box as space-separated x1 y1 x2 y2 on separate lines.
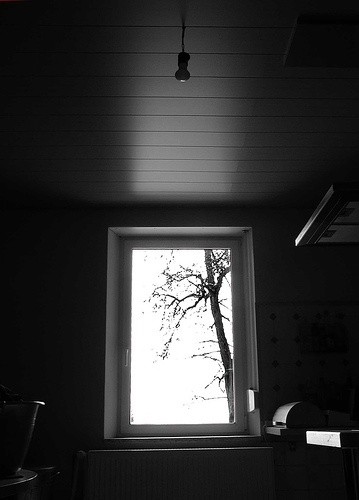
86 447 278 500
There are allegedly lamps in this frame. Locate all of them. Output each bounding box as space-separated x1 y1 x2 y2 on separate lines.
172 23 191 79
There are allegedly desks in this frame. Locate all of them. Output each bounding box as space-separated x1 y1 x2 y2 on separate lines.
265 424 358 500
